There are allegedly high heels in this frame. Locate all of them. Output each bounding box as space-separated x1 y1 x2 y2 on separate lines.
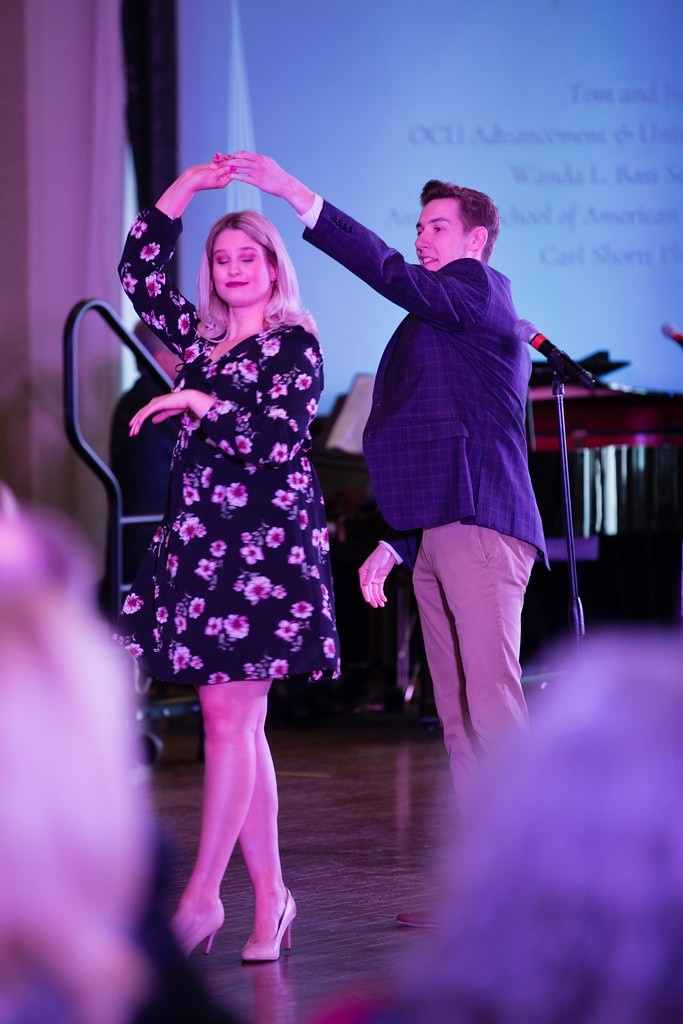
241 889 298 962
177 898 224 958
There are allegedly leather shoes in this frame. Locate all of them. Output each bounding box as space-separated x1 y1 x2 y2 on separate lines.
397 909 434 930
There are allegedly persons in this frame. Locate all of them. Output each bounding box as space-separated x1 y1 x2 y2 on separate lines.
95 318 186 626
222 150 550 930
109 155 340 962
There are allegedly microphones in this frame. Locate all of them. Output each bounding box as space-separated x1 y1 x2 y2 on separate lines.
660 321 683 347
512 318 596 389
